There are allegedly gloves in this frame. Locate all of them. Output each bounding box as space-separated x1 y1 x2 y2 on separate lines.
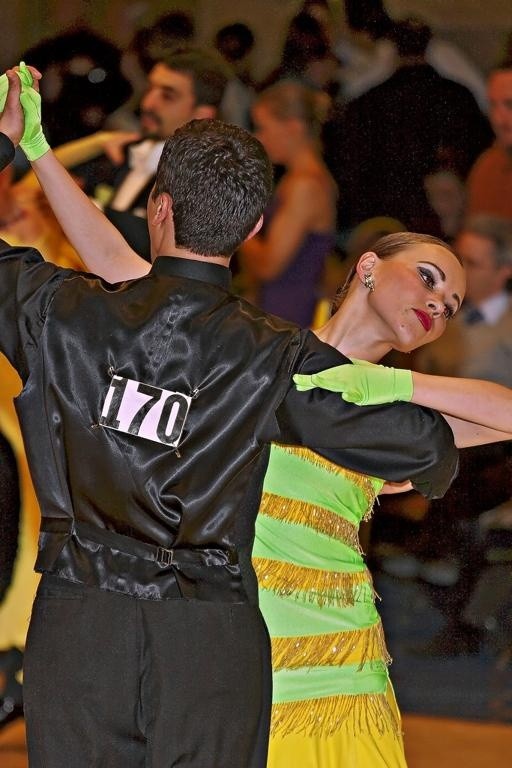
0 61 50 161
293 358 413 406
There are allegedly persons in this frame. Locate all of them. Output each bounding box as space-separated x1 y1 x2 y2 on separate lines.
0 1 512 768
